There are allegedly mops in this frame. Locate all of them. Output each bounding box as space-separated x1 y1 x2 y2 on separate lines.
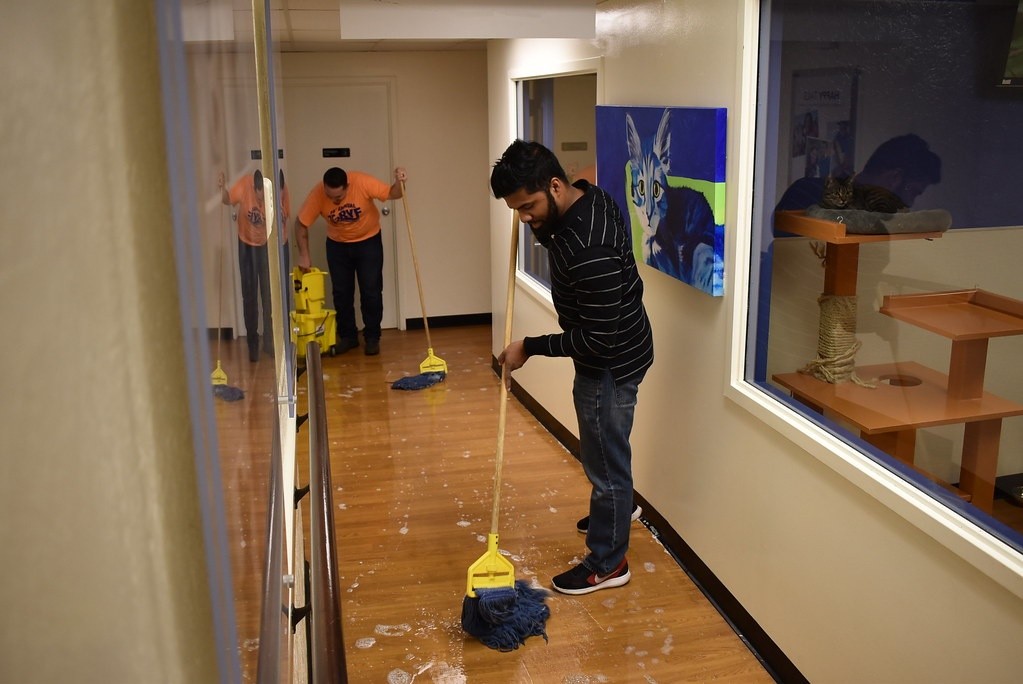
385 172 450 392
455 203 553 655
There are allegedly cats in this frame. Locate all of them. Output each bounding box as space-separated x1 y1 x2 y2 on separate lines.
822 172 910 214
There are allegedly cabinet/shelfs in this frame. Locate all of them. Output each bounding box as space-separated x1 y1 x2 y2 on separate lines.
772 208 1023 520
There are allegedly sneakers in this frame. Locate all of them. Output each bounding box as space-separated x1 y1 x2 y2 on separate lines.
550 552 631 593
575 501 643 535
333 339 360 353
364 337 380 354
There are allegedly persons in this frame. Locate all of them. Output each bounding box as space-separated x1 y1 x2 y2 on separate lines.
293 166 408 357
489 137 655 596
758 132 942 418
217 169 289 363
